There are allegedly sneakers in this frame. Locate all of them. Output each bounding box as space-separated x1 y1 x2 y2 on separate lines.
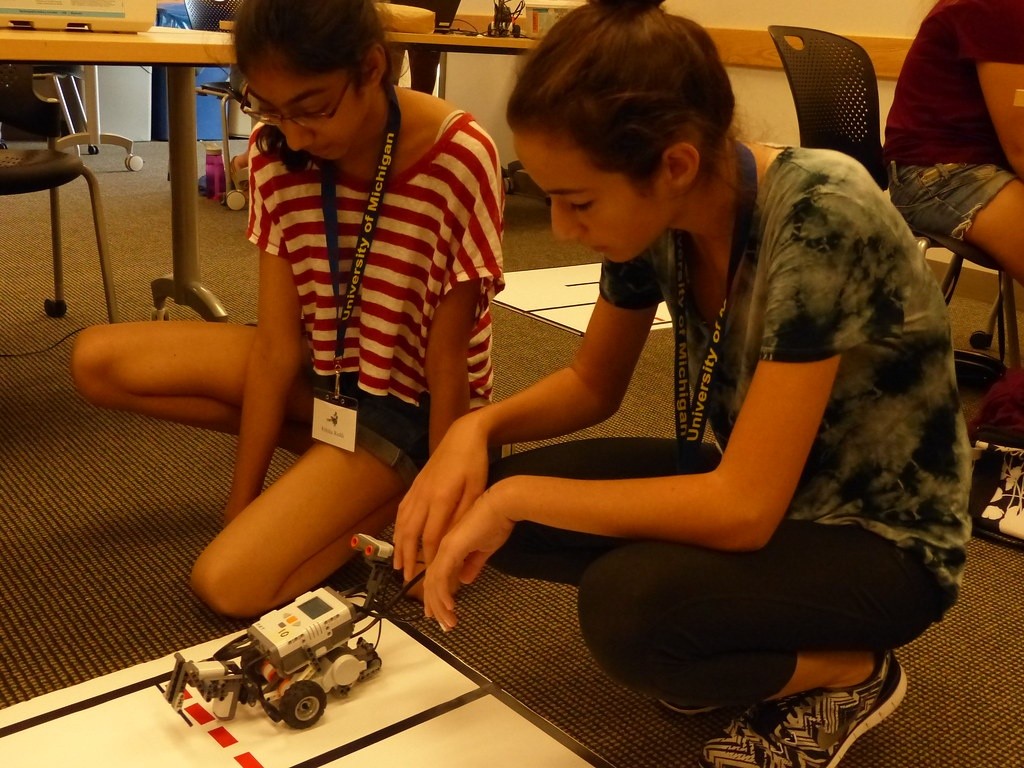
659 698 732 716
696 646 908 768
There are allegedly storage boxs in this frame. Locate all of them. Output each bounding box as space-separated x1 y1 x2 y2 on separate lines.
525 4 580 40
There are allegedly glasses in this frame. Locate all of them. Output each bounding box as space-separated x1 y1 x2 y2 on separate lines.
239 64 357 131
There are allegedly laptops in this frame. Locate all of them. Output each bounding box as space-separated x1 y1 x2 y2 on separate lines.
391 0 461 35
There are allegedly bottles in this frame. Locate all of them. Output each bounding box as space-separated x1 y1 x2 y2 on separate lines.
200 142 225 201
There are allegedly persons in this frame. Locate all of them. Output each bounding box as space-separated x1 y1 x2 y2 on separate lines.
71 0 515 617
886 0 1024 551
393 0 971 768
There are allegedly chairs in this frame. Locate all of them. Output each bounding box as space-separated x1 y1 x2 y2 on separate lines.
24 61 99 156
166 0 242 204
766 24 1021 370
0 60 120 324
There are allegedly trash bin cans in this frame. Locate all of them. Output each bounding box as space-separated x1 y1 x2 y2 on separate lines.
157 3 230 141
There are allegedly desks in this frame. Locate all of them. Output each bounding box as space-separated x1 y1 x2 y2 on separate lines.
0 27 236 323
218 21 537 53
